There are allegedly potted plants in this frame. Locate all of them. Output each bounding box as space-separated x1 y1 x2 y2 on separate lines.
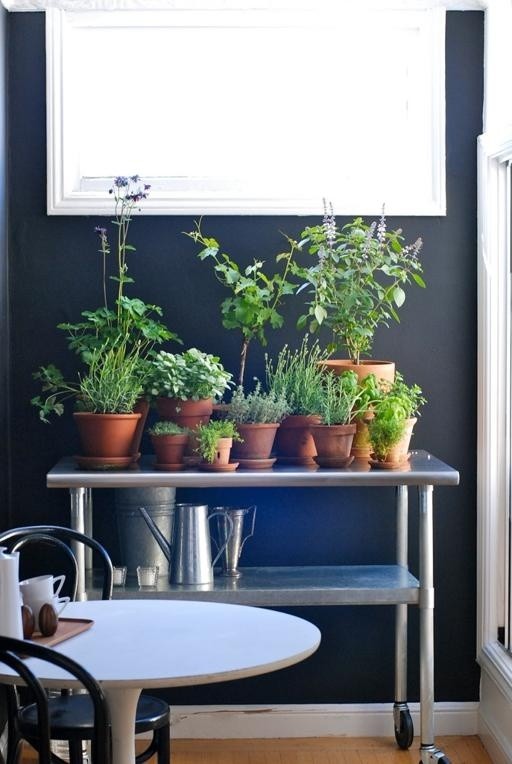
73 359 426 470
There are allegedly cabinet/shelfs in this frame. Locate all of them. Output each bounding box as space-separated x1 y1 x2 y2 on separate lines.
42 446 459 764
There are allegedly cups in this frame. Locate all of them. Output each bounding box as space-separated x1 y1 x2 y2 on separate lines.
19 572 70 629
110 565 158 588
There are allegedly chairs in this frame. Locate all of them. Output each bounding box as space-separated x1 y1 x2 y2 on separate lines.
0 634 111 764
0 521 175 764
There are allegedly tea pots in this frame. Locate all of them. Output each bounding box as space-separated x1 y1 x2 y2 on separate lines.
136 501 259 585
0 545 24 643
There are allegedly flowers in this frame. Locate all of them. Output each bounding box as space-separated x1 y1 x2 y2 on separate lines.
96 175 151 236
316 198 425 274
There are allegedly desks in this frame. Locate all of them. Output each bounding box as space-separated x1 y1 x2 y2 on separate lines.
0 592 321 764
